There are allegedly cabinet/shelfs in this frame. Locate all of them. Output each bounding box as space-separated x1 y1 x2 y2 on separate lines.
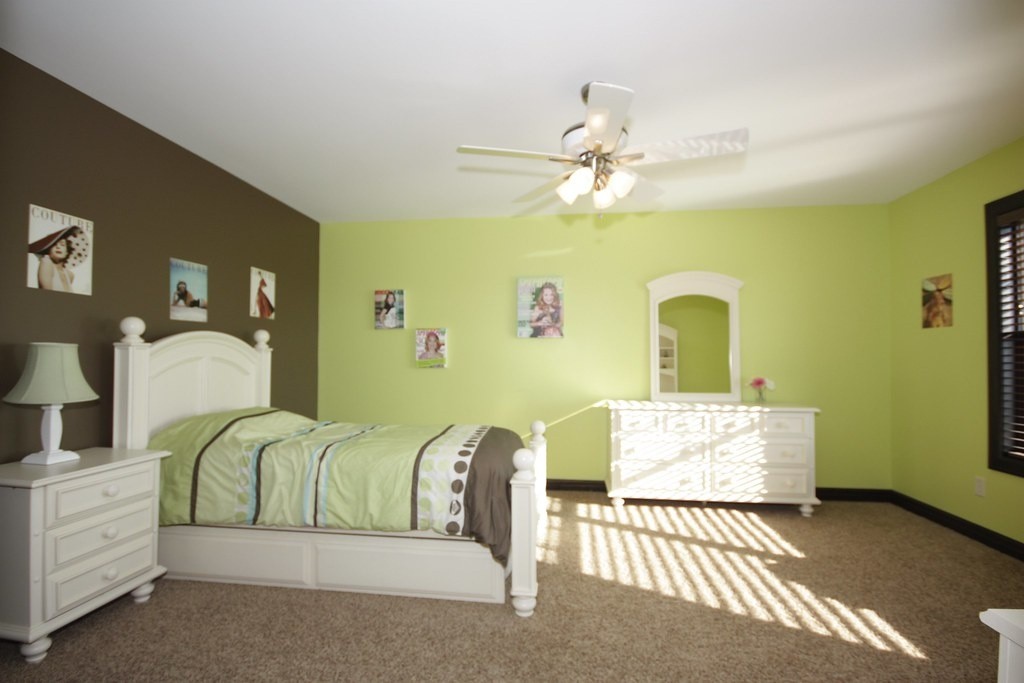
658 323 678 392
603 400 822 518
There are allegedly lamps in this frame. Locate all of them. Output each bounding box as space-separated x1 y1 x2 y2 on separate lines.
3 342 99 465
556 166 637 211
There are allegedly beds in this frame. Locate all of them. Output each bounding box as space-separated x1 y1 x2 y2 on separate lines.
111 316 545 618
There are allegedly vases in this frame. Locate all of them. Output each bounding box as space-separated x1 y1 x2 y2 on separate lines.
754 390 767 408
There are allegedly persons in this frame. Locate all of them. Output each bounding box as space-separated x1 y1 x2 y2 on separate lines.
380 292 398 328
419 332 444 367
252 270 274 318
529 283 564 338
28 226 89 293
173 282 207 309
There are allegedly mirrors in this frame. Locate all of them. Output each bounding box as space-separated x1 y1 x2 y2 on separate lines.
646 270 742 401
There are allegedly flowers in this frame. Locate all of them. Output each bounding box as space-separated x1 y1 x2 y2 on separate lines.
745 376 776 402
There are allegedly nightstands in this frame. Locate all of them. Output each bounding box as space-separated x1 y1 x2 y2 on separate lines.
1 447 174 663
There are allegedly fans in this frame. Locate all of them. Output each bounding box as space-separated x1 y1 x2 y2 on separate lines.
452 82 747 202
